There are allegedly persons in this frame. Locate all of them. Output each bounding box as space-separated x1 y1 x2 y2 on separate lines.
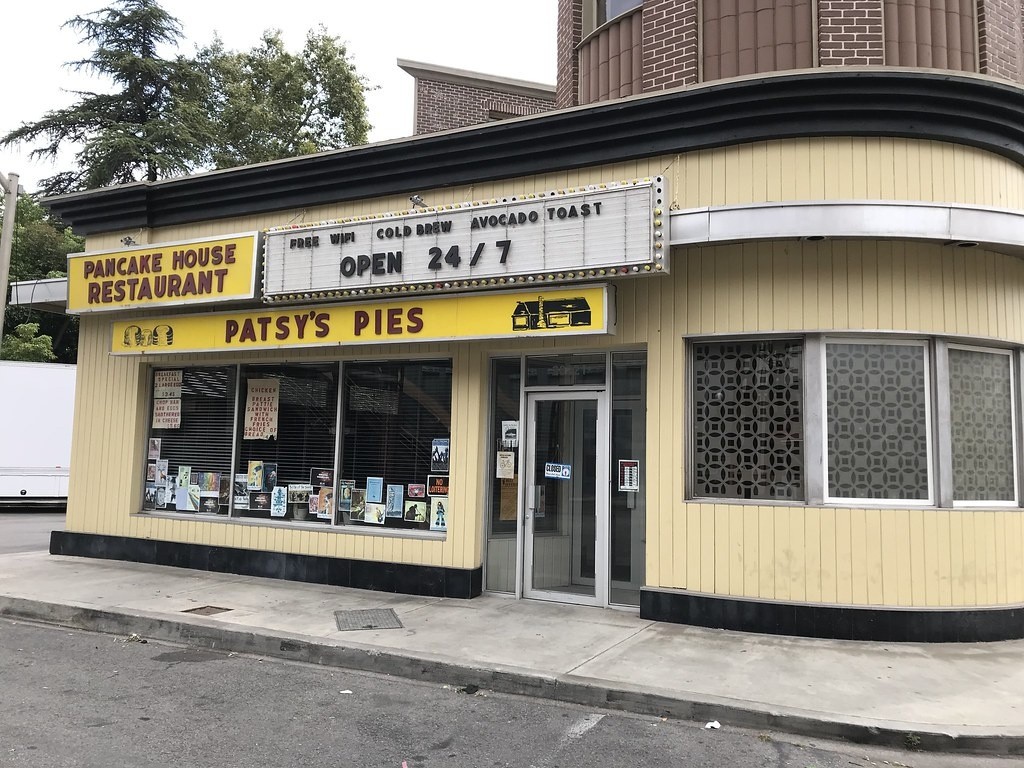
409 504 417 520
376 507 382 521
436 502 444 520
170 487 176 502
299 493 303 500
292 492 296 500
305 493 309 502
